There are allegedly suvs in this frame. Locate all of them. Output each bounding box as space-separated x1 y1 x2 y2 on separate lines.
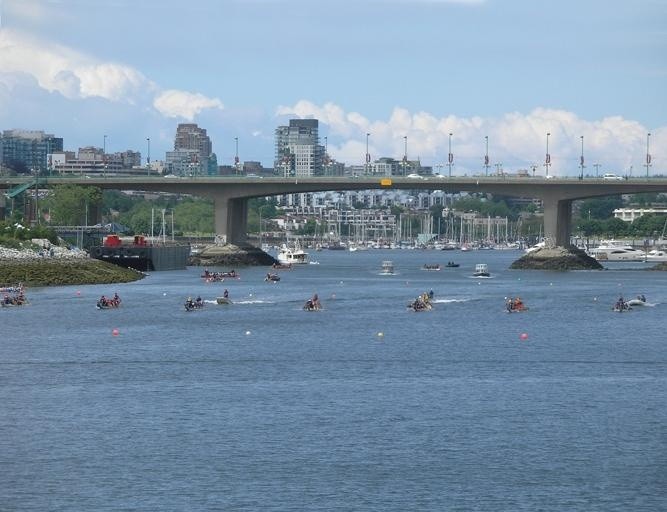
603 173 624 181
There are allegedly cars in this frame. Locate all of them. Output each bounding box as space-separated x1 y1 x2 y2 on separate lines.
406 172 424 179
246 174 259 178
163 175 179 178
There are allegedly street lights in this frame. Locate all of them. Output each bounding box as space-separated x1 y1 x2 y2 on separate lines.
646 132 653 180
482 134 490 177
578 134 586 180
365 132 371 177
403 135 408 178
232 137 240 175
144 136 151 177
543 132 551 179
47 136 52 177
323 135 330 177
102 134 108 177
447 132 455 178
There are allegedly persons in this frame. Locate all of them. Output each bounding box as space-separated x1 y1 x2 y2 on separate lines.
304 293 320 311
3 291 24 306
414 287 434 311
505 296 524 310
264 271 278 281
210 273 223 281
100 292 120 307
637 294 646 302
224 288 229 298
187 295 202 306
615 297 628 309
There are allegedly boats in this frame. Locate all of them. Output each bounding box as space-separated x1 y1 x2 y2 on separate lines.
403 289 435 315
200 267 241 283
88 205 191 272
215 294 233 305
0 285 32 309
184 296 208 312
96 298 123 310
504 295 530 315
302 299 326 312
613 298 649 313
